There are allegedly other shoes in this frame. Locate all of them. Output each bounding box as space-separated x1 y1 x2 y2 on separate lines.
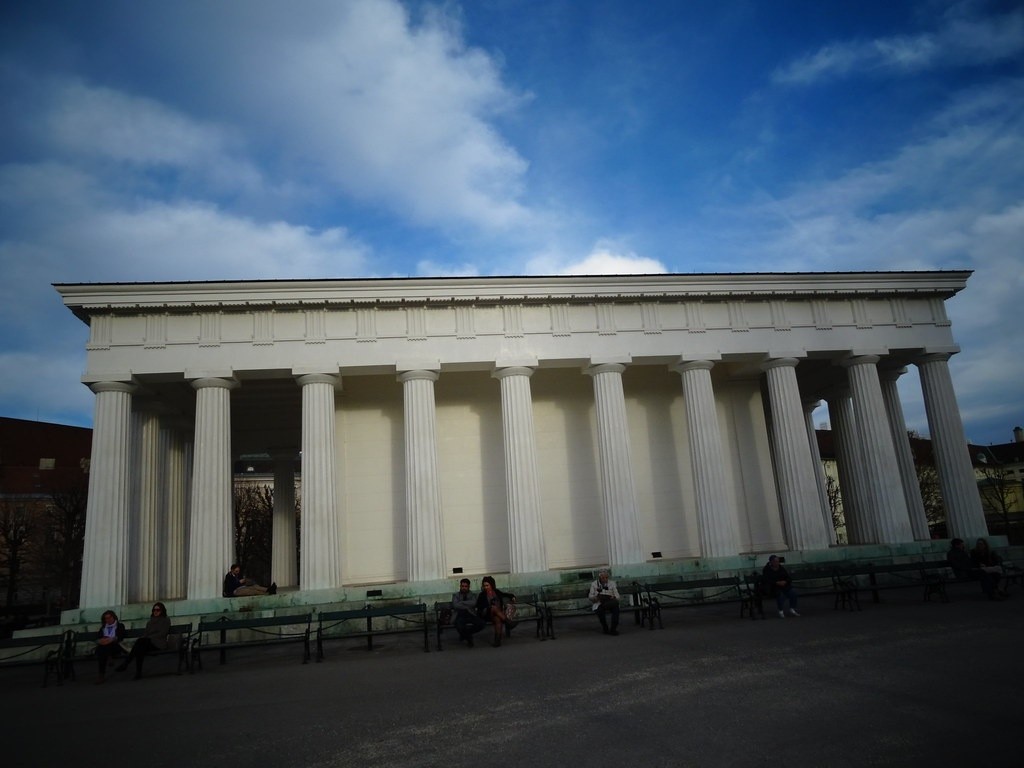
999 591 1011 597
609 630 619 635
990 594 1005 601
603 624 608 634
133 674 142 681
114 664 128 672
267 582 277 594
467 638 473 647
460 635 464 641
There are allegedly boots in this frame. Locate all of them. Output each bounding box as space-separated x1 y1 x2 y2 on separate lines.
503 618 518 630
494 633 502 647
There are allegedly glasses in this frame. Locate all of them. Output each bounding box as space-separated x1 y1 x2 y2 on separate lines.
152 609 160 612
462 585 468 588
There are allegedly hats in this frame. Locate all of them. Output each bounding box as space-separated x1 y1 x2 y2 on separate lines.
951 538 963 545
769 555 776 561
483 576 495 589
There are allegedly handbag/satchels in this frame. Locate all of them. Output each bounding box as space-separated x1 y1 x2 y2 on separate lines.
505 599 516 620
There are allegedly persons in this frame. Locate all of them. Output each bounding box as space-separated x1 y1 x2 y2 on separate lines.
56 592 76 624
114 602 171 679
763 554 801 617
947 538 1005 601
223 564 277 597
588 569 621 635
96 610 125 682
452 576 518 648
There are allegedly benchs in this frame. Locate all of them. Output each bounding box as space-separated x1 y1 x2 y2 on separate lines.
0 546 1024 686
